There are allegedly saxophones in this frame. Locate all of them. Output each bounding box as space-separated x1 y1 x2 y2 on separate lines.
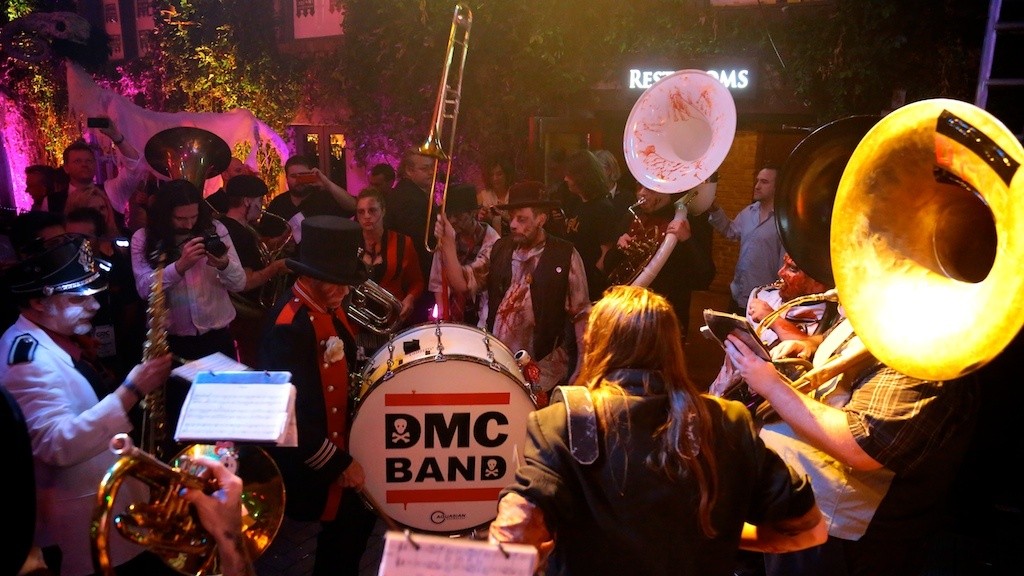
134 253 172 457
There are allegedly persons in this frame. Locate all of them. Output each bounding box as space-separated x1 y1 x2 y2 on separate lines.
25 116 149 382
476 155 519 237
203 154 501 576
708 161 788 341
479 183 590 393
130 179 245 362
537 147 635 301
710 251 968 576
0 385 256 576
603 174 718 346
0 209 181 576
488 283 828 576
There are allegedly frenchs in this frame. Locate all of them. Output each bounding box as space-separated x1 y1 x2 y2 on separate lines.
608 71 737 291
146 126 232 198
755 95 1024 422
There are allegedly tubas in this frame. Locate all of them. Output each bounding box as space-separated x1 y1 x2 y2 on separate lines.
257 210 404 333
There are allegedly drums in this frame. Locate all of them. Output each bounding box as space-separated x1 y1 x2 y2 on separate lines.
350 320 539 533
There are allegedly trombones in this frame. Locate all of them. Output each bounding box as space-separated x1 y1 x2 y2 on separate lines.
418 1 473 253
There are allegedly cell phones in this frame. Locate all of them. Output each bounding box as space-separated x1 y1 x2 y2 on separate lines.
296 173 317 183
87 117 109 128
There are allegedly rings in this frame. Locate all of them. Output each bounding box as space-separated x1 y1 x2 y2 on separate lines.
670 225 673 229
752 308 755 312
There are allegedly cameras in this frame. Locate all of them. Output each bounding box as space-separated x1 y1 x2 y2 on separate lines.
202 233 227 258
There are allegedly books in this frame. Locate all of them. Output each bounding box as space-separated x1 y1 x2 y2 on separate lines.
174 384 297 446
702 308 772 363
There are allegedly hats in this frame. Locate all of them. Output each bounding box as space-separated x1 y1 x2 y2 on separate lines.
226 174 268 197
284 215 369 285
7 232 112 296
495 180 562 211
435 185 482 212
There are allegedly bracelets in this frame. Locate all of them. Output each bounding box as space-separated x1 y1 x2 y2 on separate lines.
113 134 125 145
123 379 145 400
